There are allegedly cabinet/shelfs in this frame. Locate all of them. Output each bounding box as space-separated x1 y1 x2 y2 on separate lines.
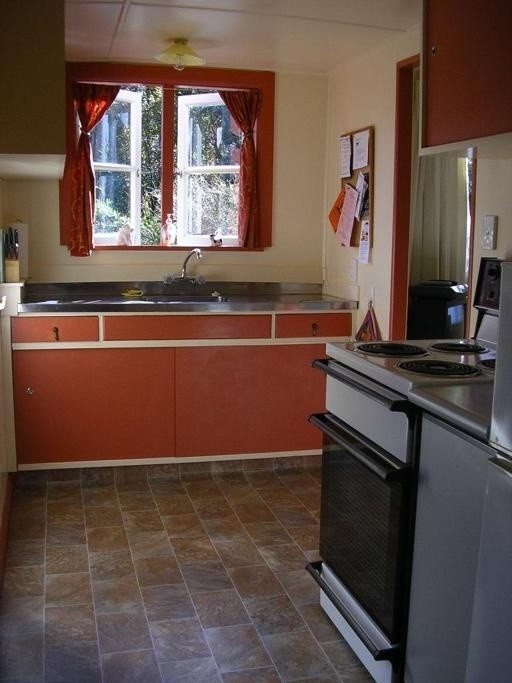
9 309 355 469
421 0 511 159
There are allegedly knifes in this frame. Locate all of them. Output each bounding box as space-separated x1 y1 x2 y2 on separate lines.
2 227 21 258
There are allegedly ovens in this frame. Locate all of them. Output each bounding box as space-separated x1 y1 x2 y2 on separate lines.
305 352 407 683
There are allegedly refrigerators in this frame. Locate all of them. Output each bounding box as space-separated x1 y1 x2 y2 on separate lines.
461 260 510 683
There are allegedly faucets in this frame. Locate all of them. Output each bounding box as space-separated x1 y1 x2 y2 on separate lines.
180 248 203 279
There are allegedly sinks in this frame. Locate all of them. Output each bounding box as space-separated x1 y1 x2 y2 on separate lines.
123 295 234 303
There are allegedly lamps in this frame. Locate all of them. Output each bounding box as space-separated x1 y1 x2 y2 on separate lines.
154 38 208 70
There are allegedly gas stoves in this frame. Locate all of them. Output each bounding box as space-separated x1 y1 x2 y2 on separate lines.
325 335 496 394
